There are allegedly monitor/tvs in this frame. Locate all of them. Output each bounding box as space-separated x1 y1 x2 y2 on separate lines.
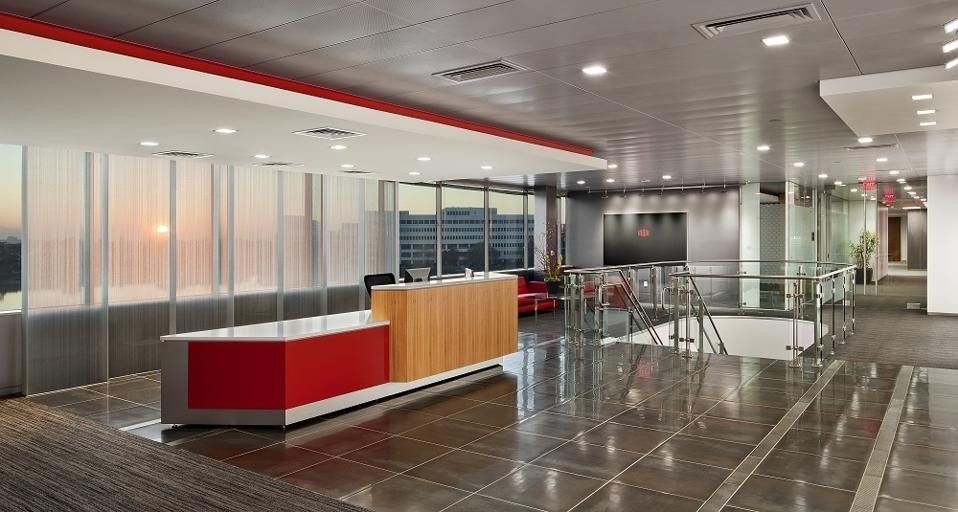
404 268 430 282
465 268 474 277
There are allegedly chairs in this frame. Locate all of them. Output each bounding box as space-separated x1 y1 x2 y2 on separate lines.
364 272 396 298
518 274 559 314
577 275 633 311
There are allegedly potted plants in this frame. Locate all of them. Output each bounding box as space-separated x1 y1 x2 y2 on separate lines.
533 220 564 294
846 227 881 285
